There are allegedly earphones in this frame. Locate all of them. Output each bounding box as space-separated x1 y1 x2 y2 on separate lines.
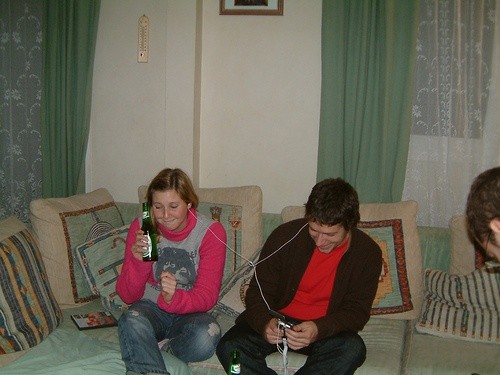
188 204 191 209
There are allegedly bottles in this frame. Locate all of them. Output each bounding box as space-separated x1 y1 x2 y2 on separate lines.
140 202 158 262
228 349 242 375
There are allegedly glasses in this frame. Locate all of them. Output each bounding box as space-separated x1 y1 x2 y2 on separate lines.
483 231 500 274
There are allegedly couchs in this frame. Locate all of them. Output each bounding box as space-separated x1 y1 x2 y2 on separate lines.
0 200 500 375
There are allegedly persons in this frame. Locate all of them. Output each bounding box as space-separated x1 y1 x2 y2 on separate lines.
117 168 227 375
467 167 500 262
216 177 382 375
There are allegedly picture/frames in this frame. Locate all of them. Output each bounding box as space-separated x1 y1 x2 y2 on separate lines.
219 0 284 16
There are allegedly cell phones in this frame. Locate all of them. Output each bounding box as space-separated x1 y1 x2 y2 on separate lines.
270 309 294 329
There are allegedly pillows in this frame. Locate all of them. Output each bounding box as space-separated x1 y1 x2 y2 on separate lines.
76 225 131 316
216 248 262 316
0 214 64 353
282 202 422 318
29 188 125 307
138 185 263 289
450 214 484 276
416 268 500 346
